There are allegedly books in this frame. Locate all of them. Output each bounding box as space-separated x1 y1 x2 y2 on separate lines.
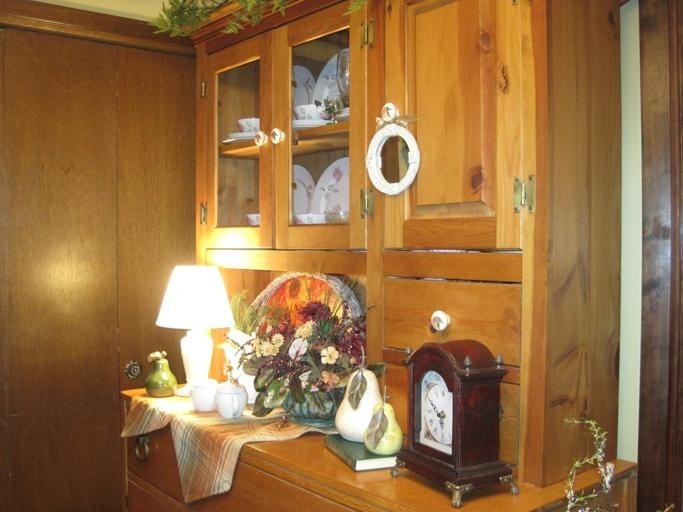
323 433 398 473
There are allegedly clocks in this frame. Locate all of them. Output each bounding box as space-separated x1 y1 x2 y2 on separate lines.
402 338 518 509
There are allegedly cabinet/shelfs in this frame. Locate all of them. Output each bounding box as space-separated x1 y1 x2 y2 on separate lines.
120 385 639 512
188 1 368 249
370 1 623 488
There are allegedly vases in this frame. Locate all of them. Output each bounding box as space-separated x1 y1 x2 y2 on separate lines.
280 394 340 434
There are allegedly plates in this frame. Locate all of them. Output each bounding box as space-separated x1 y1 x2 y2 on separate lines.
311 153 350 220
312 46 349 117
291 65 316 120
227 132 260 138
294 164 315 213
291 120 331 127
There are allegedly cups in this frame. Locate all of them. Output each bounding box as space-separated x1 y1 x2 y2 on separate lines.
190 379 217 412
216 383 249 418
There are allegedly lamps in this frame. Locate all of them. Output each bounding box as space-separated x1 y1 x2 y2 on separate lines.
154 265 235 396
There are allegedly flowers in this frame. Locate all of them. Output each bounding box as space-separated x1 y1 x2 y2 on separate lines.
229 264 383 416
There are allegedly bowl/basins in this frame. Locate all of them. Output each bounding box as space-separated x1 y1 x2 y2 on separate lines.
246 213 260 225
294 104 324 119
294 214 326 224
238 118 260 132
